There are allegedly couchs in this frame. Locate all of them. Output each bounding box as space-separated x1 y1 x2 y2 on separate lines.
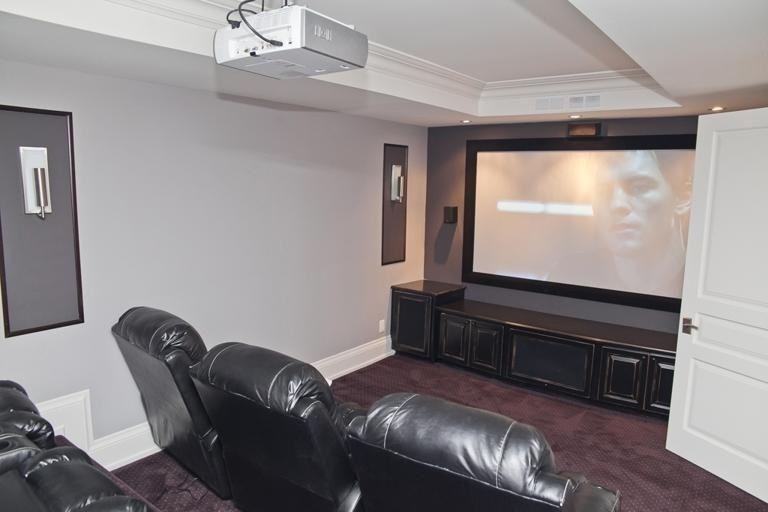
110 307 230 501
0 447 157 512
0 380 55 449
346 389 621 511
188 342 362 512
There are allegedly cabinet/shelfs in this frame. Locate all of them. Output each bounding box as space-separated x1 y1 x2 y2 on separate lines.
391 280 467 362
433 298 678 422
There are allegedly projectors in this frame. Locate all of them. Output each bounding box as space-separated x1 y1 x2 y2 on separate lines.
213 4 369 81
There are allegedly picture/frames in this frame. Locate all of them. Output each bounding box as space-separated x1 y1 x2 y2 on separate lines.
382 142 409 266
0 105 85 338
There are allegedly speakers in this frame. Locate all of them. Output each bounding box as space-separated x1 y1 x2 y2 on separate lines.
444 206 458 224
